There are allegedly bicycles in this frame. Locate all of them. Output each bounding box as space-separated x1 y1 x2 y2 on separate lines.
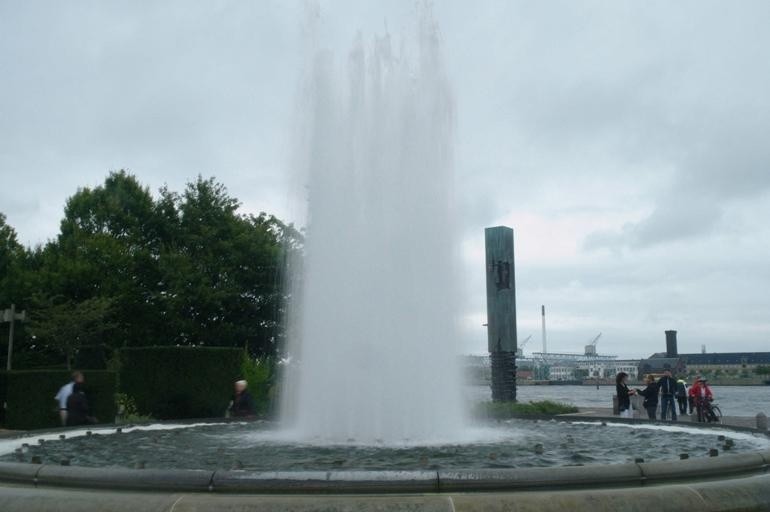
663 393 674 422
697 395 723 423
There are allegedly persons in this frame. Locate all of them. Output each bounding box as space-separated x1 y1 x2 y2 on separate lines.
615 372 636 418
637 375 658 419
225 379 258 417
675 375 719 423
657 368 677 420
54 372 85 425
66 381 95 425
